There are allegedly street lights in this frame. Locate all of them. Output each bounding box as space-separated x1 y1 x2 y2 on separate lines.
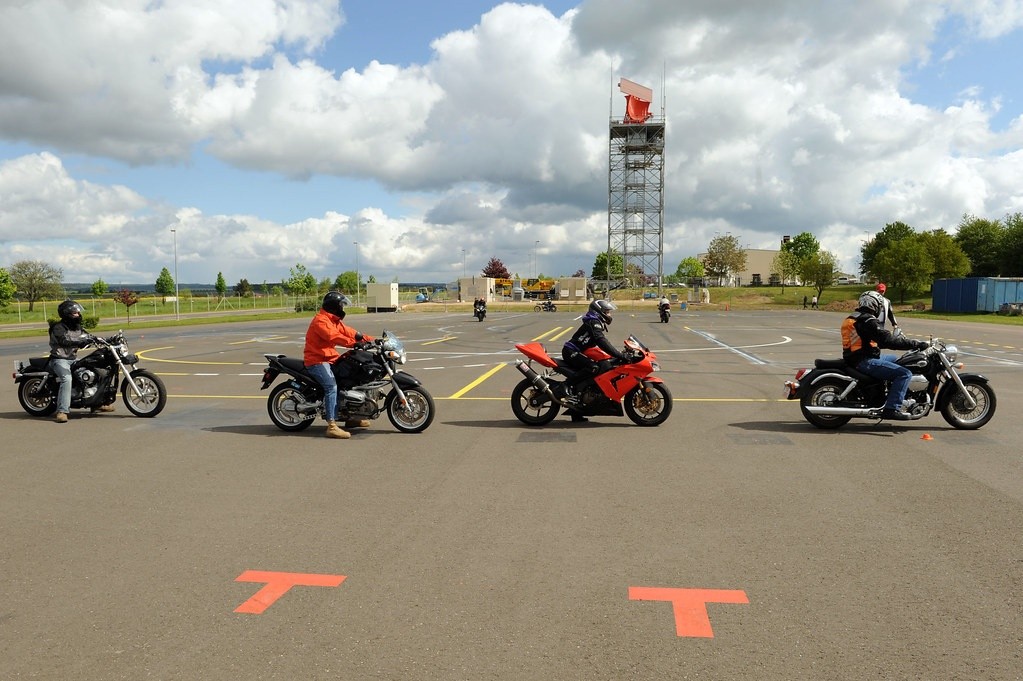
535 240 540 278
170 229 179 320
864 230 869 246
462 249 465 276
354 242 360 307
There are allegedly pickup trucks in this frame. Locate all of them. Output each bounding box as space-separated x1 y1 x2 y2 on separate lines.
837 277 849 285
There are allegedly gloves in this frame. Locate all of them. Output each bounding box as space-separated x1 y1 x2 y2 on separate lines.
621 352 632 363
105 335 113 342
77 337 94 349
374 338 383 344
916 342 928 352
353 341 369 352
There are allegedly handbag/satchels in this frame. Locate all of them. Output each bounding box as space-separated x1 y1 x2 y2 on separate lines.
893 327 906 341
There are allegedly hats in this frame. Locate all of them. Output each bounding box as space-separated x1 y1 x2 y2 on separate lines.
876 284 886 293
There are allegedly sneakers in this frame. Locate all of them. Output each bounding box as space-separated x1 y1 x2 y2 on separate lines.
99 404 115 412
56 412 67 422
880 408 911 419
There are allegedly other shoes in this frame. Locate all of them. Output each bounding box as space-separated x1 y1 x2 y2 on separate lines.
570 413 588 423
592 389 608 404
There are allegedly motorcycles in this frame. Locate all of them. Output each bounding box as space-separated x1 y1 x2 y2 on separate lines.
509 333 674 427
784 326 998 430
542 302 557 312
260 328 436 434
475 306 487 322
11 328 167 417
657 303 671 323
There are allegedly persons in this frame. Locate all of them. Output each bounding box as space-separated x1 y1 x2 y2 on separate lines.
48 300 115 422
841 290 929 420
811 295 818 308
876 284 898 330
660 295 671 317
473 297 487 318
803 296 808 309
550 287 555 301
562 299 629 424
304 292 383 438
587 289 590 300
603 288 607 299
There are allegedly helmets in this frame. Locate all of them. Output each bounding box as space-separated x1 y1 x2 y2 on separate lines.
858 290 884 316
663 295 666 298
588 299 613 325
322 292 347 320
58 300 82 329
480 298 484 302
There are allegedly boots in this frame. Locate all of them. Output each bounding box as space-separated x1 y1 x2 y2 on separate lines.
326 421 351 439
345 416 370 427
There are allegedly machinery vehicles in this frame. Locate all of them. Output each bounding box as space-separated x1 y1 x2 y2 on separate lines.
494 277 557 296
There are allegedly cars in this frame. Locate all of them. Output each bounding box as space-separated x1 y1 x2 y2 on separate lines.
416 287 445 302
